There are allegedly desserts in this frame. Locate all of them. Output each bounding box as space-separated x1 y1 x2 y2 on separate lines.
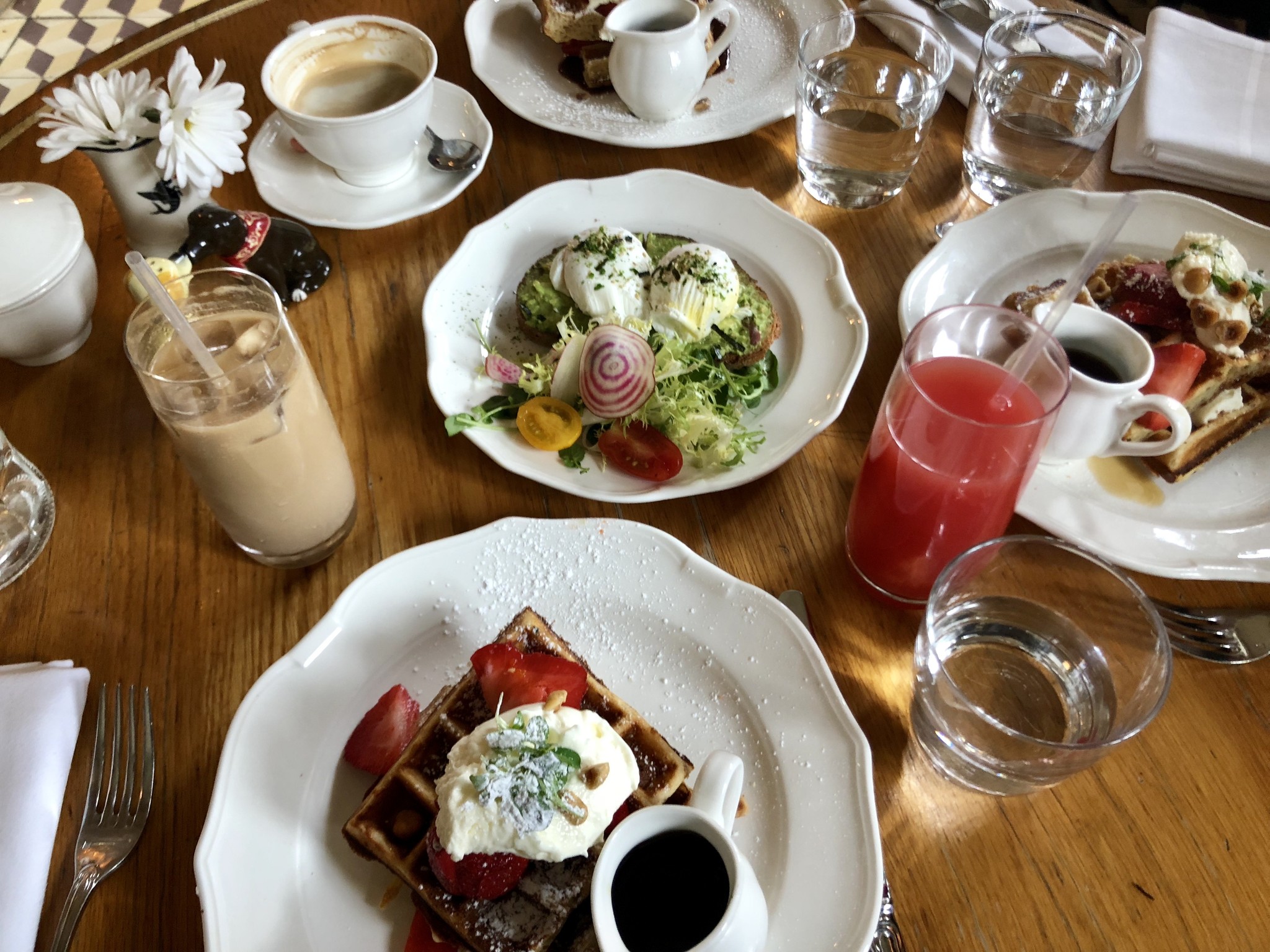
435 700 641 864
1167 230 1251 359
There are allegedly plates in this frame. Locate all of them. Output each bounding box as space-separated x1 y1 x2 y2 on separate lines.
248 71 493 232
422 166 870 504
190 512 884 952
896 187 1270 592
461 1 855 149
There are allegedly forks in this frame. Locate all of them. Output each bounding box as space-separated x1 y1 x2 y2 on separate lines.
1134 573 1270 665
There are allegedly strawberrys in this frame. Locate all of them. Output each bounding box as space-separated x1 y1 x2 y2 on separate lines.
1137 342 1208 430
342 683 420 776
470 642 588 715
426 820 530 899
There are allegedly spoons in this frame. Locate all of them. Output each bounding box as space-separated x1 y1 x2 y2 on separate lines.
975 1 1051 35
42 679 154 952
423 122 482 174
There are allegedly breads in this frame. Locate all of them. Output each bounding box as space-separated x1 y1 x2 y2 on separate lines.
345 607 693 952
1006 230 1270 484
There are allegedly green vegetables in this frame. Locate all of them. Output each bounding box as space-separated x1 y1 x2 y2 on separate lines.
444 223 784 477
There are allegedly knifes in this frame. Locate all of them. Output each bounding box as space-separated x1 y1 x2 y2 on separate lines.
921 1 1055 54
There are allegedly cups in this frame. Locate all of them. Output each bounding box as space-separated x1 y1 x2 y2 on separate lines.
591 750 769 951
261 14 440 188
128 262 355 573
1025 302 1190 466
962 8 1143 209
0 423 57 589
0 179 98 366
843 305 1069 607
794 12 954 211
604 0 741 124
912 533 1172 798
